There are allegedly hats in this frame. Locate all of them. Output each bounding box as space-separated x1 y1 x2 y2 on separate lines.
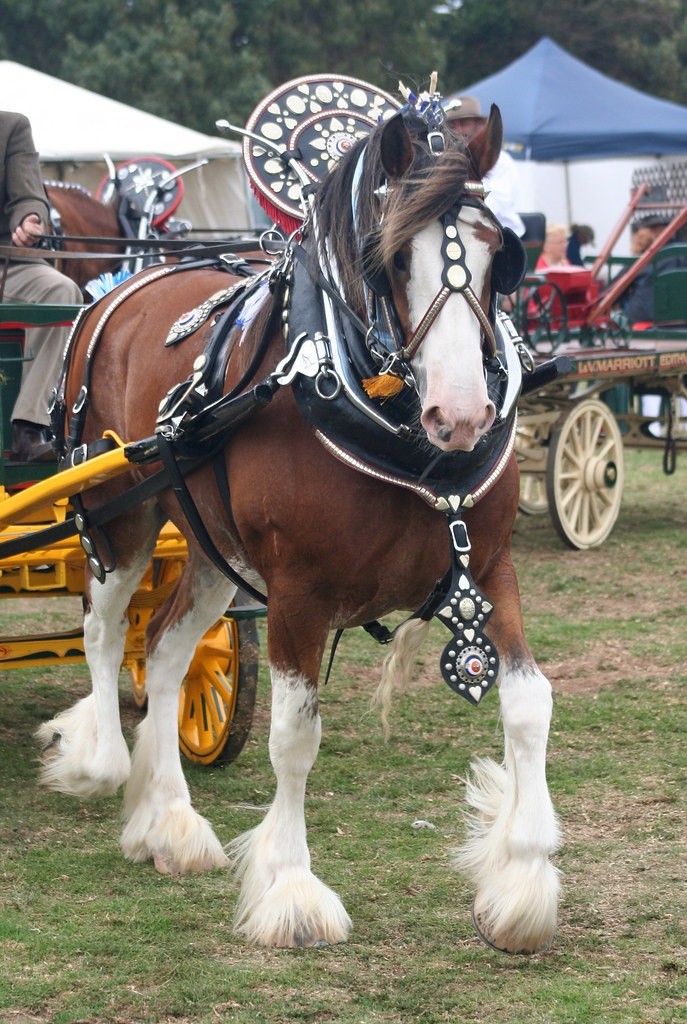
578 225 596 248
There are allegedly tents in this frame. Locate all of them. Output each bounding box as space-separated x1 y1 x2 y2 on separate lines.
0 35 687 286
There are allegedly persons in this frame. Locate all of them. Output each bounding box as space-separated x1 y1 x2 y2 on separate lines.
0 111 83 463
500 224 593 312
602 215 687 310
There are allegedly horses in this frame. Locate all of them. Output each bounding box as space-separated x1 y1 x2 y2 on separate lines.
25 68 570 952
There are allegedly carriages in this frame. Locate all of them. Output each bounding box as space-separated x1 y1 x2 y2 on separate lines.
1 92 573 958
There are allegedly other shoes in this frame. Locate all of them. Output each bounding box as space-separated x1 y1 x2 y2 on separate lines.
9 421 57 462
